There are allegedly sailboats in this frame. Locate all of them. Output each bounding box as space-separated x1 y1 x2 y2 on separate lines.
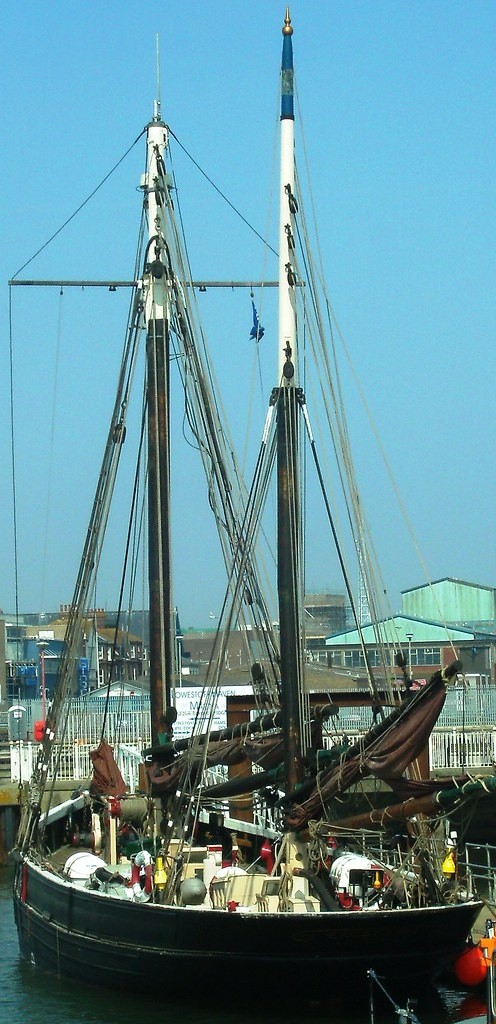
0 6 496 1024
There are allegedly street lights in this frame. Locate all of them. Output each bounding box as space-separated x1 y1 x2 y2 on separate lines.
406 633 414 680
36 641 50 722
174 634 184 687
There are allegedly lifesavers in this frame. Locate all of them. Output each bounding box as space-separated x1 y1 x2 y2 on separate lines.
330 854 392 911
131 850 156 903
208 866 249 903
